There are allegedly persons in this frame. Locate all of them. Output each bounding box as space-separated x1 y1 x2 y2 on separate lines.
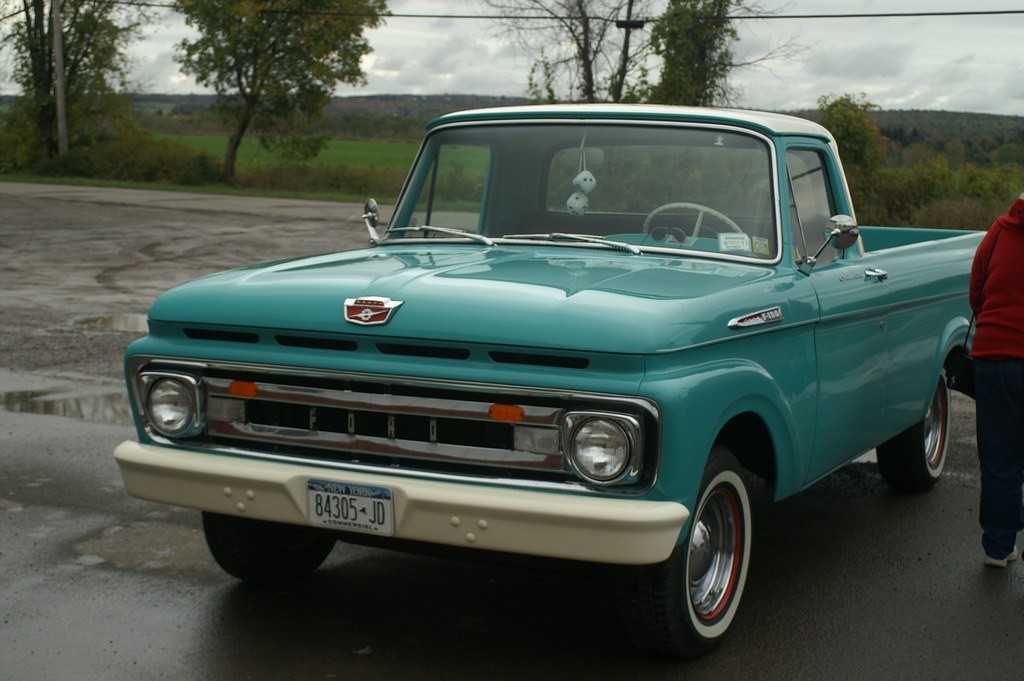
968 193 1024 568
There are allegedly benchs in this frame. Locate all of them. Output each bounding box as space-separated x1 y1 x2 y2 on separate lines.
518 210 835 263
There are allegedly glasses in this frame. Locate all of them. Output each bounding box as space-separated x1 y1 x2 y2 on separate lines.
640 226 685 248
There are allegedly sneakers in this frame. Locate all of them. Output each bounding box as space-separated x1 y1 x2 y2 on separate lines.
982 546 1018 568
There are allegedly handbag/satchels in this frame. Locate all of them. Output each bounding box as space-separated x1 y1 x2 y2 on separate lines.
946 345 978 399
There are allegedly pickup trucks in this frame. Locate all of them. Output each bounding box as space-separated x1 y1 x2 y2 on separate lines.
109 99 991 652
